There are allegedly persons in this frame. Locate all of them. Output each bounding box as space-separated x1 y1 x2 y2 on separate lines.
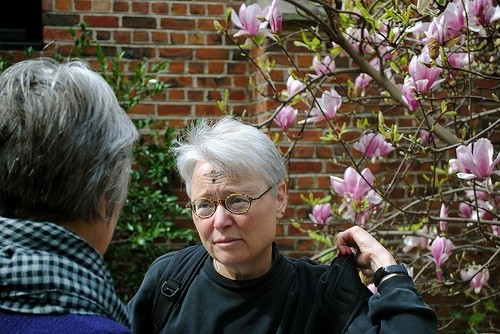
126 111 439 334
1 56 143 334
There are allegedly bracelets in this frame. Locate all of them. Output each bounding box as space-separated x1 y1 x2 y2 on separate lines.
373 263 409 288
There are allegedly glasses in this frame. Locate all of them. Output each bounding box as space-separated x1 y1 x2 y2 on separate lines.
185 180 280 218
